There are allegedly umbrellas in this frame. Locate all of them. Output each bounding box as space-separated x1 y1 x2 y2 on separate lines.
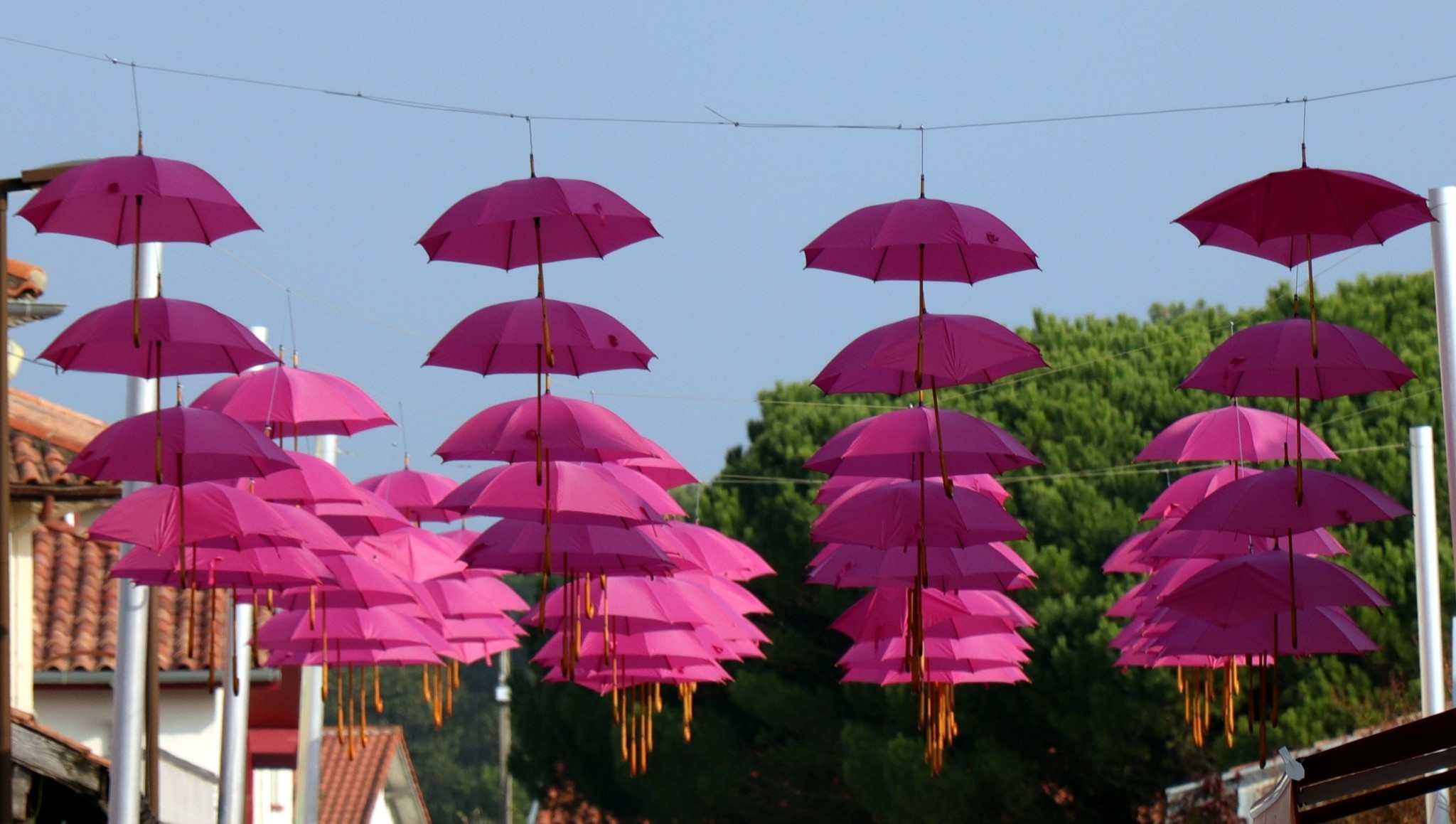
414 153 666 367
31 273 532 764
428 269 661 485
1170 141 1443 359
1170 294 1421 507
12 130 263 348
803 392 1039 778
797 174 1044 387
430 371 777 779
1103 398 1418 769
806 304 1053 498
